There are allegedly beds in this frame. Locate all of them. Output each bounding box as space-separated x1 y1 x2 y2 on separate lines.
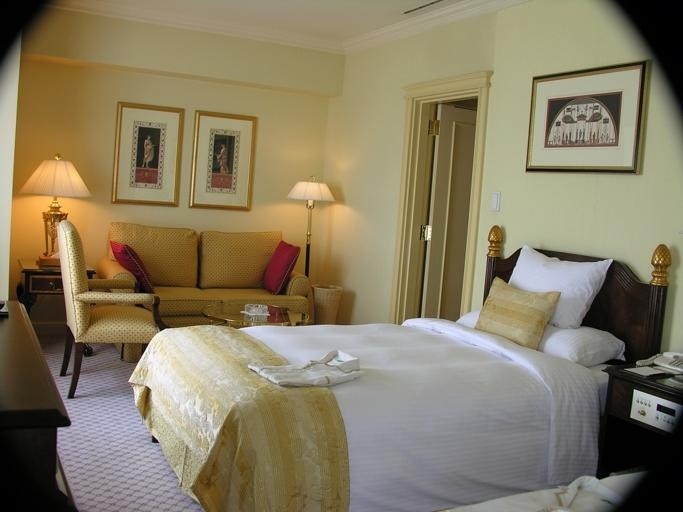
128 225 670 512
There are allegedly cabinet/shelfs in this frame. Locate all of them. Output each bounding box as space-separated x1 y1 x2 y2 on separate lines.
14 263 96 317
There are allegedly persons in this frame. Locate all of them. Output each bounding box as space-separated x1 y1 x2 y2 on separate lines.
142 135 153 168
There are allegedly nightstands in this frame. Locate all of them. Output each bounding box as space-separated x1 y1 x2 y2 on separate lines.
603 361 682 470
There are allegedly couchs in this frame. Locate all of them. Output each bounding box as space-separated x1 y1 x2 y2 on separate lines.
96 220 311 362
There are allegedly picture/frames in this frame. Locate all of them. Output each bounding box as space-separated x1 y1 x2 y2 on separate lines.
524 58 648 174
189 110 259 212
109 99 184 207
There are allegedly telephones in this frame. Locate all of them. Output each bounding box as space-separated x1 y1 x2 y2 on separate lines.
654 352 683 371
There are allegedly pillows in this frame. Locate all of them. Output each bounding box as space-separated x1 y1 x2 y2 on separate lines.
110 240 155 294
263 238 300 293
267 305 289 322
508 244 613 328
476 275 562 352
455 306 626 368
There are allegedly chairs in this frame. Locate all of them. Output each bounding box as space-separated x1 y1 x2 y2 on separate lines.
55 219 160 396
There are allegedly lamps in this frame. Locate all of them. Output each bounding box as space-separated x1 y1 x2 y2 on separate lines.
286 176 336 277
18 154 92 265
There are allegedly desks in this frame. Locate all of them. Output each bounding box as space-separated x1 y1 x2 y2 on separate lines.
0 297 76 510
